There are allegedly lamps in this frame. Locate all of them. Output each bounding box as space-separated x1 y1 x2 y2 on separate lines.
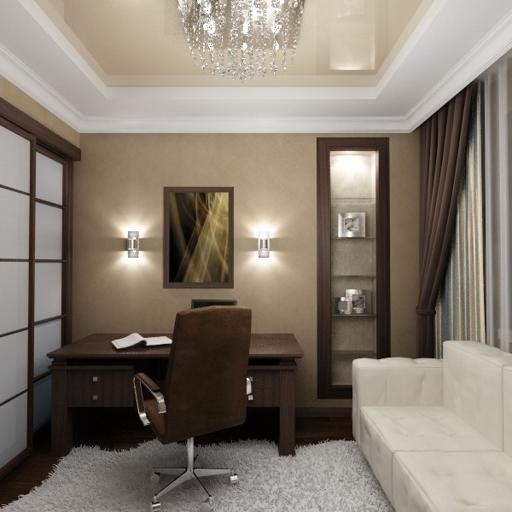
128 230 139 258
258 231 270 259
175 0 306 82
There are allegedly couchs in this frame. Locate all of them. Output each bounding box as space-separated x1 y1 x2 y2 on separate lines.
351 339 512 512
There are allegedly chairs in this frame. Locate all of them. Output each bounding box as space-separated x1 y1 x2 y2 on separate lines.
132 305 254 512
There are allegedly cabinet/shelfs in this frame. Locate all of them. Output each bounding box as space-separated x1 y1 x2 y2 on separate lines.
316 137 391 400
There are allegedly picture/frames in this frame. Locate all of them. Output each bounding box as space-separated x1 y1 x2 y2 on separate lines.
338 210 366 238
162 186 235 289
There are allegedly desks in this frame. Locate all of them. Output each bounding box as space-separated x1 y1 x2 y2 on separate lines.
47 333 304 455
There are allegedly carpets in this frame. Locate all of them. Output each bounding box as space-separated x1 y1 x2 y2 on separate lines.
0 438 395 512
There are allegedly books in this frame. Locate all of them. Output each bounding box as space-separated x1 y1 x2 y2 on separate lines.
111 333 173 349
